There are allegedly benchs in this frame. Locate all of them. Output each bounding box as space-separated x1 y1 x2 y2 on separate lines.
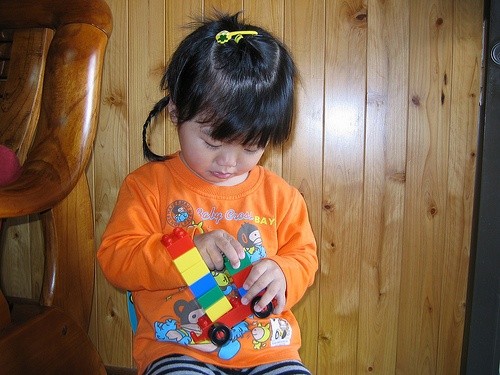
0 0 115 375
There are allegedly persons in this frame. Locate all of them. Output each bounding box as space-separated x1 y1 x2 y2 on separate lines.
97 4 318 375
